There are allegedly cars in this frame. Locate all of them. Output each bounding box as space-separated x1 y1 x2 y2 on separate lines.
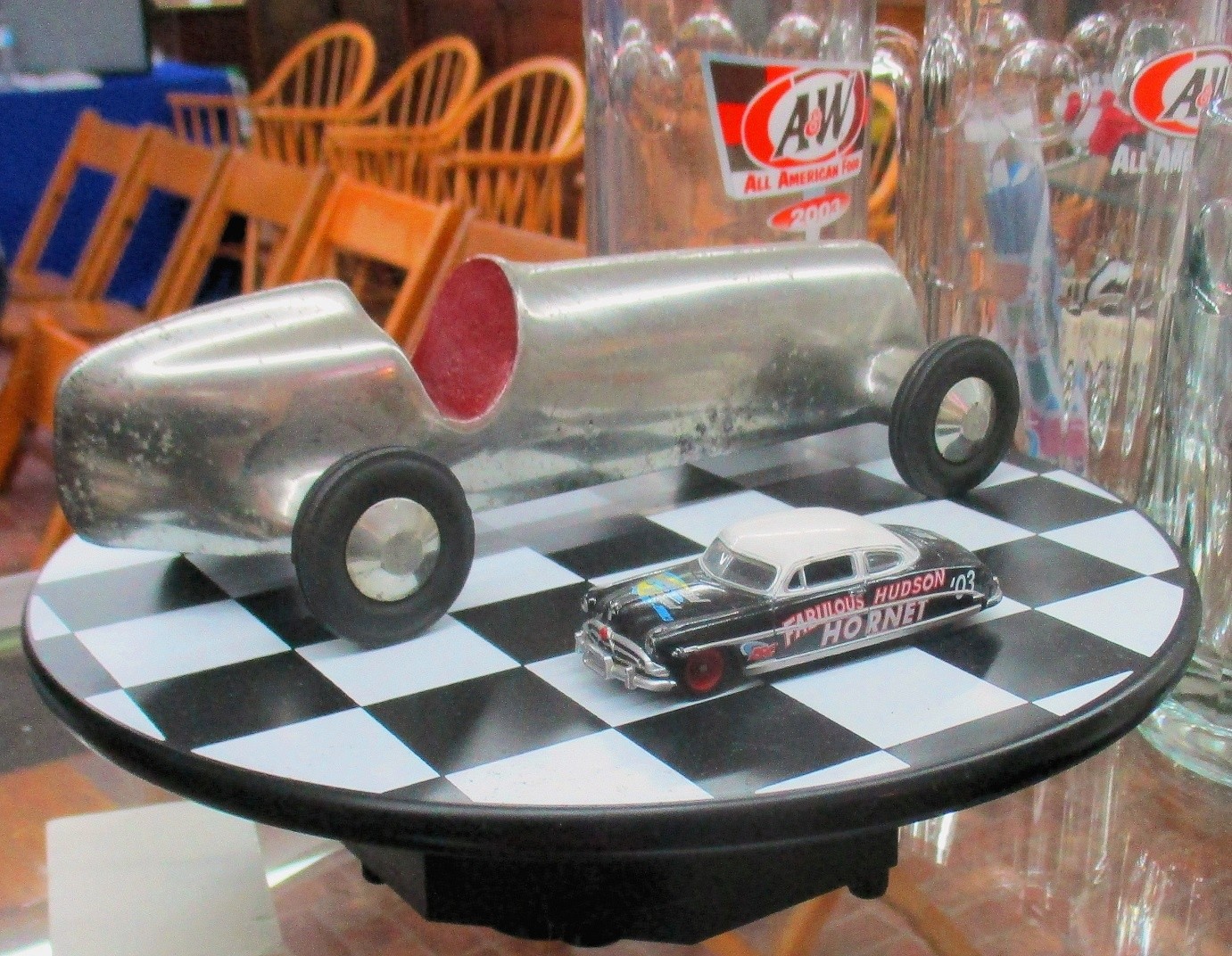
574 506 1003 697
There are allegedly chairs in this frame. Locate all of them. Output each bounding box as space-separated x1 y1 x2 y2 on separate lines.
0 25 1114 573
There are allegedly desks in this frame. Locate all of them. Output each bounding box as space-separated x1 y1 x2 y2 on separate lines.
24 437 1203 946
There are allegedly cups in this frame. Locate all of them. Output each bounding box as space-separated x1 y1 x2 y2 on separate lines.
583 0 873 259
893 0 1232 507
0 28 16 94
1130 96 1232 791
